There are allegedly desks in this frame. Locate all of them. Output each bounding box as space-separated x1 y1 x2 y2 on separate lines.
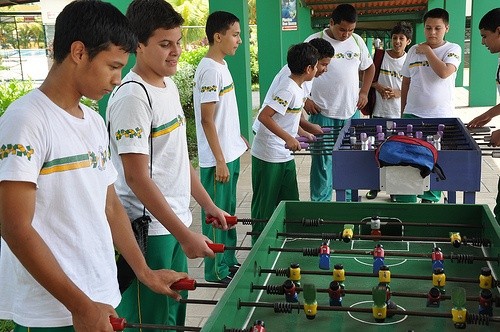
332 117 481 204
199 200 500 332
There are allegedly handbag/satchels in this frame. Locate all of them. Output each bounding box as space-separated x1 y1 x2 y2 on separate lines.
360 48 385 115
117 215 152 279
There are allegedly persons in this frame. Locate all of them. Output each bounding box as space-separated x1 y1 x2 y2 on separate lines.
390 8 462 203
192 11 250 284
373 36 381 49
252 43 319 249
252 38 334 136
467 8 500 224
105 0 238 332
303 5 375 201
365 21 413 199
0 0 190 332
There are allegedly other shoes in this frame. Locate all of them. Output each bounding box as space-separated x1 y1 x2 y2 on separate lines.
366 190 377 200
205 264 241 283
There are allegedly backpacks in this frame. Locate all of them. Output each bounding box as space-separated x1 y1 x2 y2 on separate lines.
374 135 448 196
